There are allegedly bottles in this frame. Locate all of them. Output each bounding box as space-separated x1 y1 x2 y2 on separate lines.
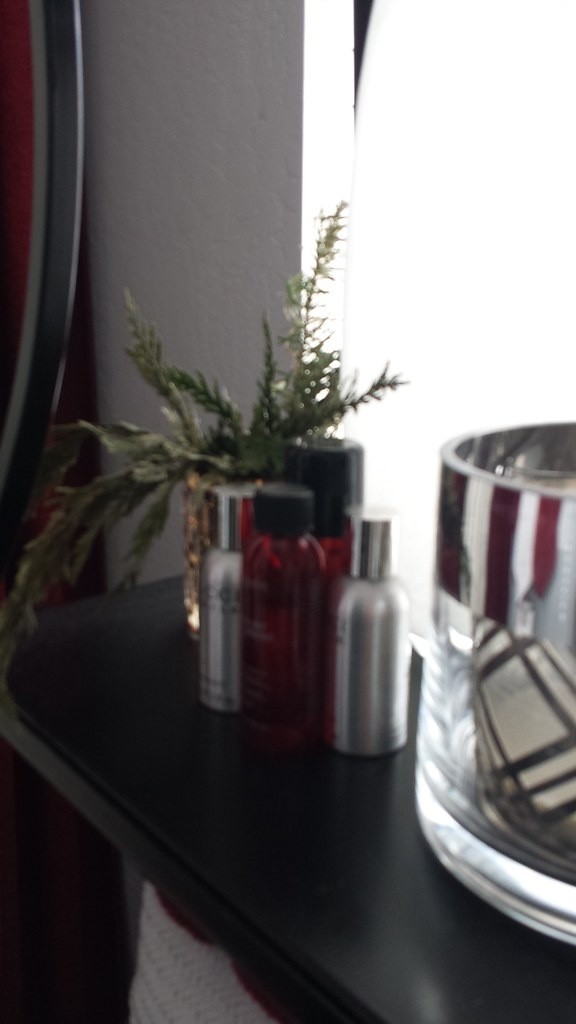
325 506 415 757
284 433 368 720
198 482 247 713
242 481 324 749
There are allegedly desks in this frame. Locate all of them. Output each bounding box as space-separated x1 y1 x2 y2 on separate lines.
2 574 576 1022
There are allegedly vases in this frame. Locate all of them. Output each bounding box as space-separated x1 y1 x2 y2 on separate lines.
184 470 268 643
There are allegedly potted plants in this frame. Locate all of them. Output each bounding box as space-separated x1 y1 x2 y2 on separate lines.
15 196 407 644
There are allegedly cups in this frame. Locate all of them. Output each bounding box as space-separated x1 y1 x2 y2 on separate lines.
414 418 576 946
